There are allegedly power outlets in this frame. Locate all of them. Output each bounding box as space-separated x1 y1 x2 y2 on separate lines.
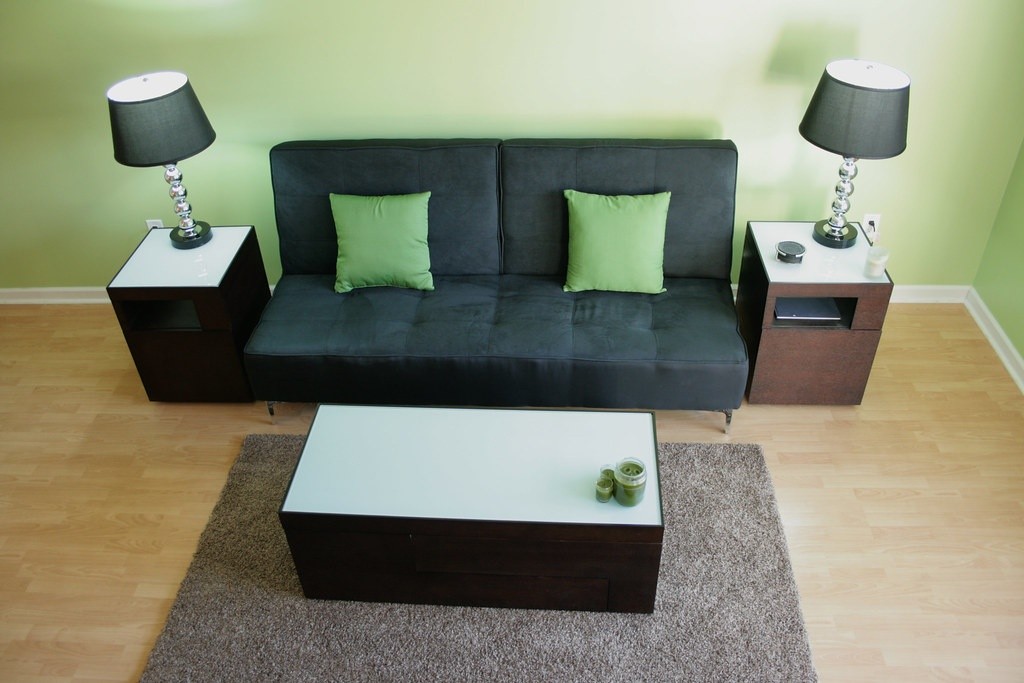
862 214 880 245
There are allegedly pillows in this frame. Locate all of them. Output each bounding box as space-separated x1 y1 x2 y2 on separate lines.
562 188 673 293
329 191 435 293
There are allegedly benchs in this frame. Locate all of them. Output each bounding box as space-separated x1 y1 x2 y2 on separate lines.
242 139 750 434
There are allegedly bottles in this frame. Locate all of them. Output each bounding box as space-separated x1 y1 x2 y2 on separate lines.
599 464 615 481
595 476 614 503
614 456 647 506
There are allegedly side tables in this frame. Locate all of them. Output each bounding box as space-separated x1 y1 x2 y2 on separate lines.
735 221 895 407
106 224 271 403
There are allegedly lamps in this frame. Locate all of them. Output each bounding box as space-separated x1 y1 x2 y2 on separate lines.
799 59 910 249
106 71 217 250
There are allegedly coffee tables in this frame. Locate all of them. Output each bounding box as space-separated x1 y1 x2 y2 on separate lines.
278 403 664 616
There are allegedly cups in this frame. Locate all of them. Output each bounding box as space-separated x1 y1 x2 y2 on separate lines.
864 246 891 279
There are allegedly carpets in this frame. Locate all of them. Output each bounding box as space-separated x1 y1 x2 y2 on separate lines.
134 434 819 682
